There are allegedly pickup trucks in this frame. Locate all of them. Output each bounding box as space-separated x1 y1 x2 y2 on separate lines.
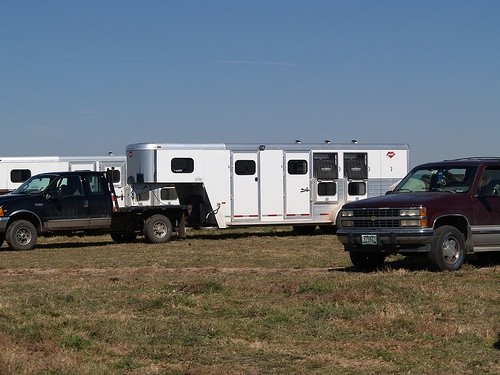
0 168 194 251
336 156 500 272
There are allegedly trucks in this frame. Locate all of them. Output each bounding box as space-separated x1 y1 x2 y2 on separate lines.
126 144 410 230
0 156 181 205
